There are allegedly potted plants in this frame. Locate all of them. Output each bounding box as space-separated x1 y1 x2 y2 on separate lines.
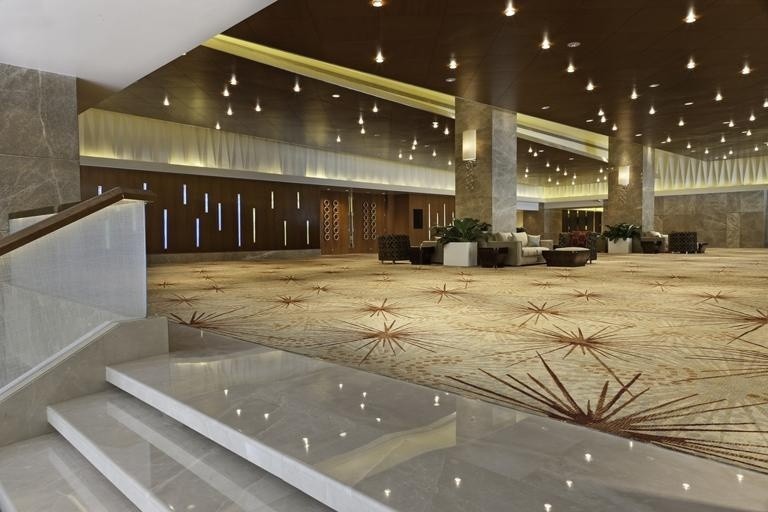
442 217 485 267
599 222 641 254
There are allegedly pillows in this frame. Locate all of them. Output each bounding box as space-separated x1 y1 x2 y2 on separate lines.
494 231 541 247
647 230 662 238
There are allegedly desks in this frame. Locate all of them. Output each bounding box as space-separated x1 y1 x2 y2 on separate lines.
478 247 508 268
542 246 590 267
409 246 435 265
641 237 658 254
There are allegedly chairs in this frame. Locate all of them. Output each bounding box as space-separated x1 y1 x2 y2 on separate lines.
377 234 410 264
420 237 443 263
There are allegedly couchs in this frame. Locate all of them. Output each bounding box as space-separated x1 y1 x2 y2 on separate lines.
487 236 554 267
642 231 669 251
667 231 697 255
554 231 597 260
